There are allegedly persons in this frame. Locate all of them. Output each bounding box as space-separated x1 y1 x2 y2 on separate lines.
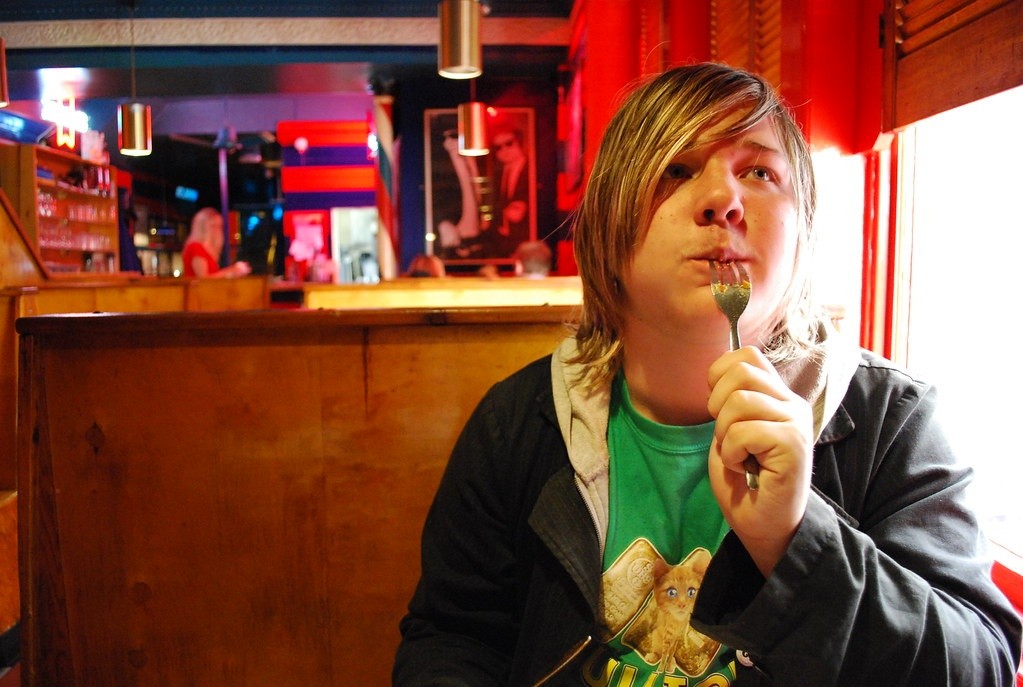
182 208 249 280
405 239 553 278
390 63 1023 687
484 121 530 258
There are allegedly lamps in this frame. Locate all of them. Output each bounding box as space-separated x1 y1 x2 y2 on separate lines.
437 0 482 80
457 77 489 156
118 16 152 156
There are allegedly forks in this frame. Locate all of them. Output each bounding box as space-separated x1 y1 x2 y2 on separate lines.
707 258 758 491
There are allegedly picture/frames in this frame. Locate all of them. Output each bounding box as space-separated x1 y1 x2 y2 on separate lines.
394 70 560 272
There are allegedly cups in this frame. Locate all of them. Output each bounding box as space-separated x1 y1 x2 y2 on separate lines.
38 187 116 273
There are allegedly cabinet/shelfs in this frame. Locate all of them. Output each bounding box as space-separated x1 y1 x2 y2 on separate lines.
5 145 120 274
